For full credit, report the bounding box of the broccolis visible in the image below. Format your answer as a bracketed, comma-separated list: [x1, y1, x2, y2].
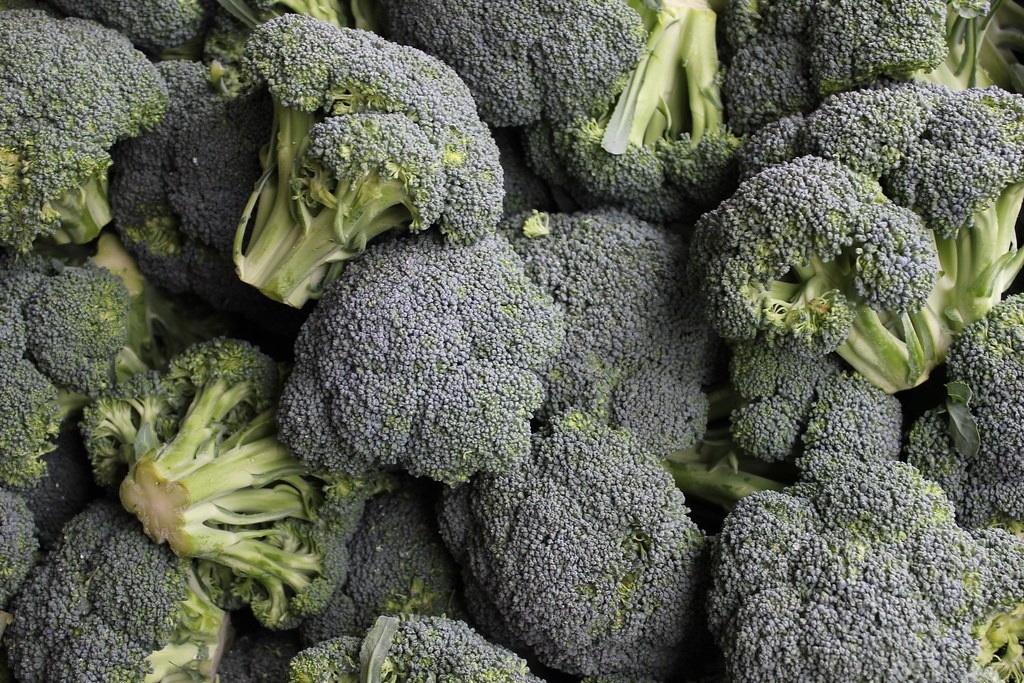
[1, 0, 1024, 683]
